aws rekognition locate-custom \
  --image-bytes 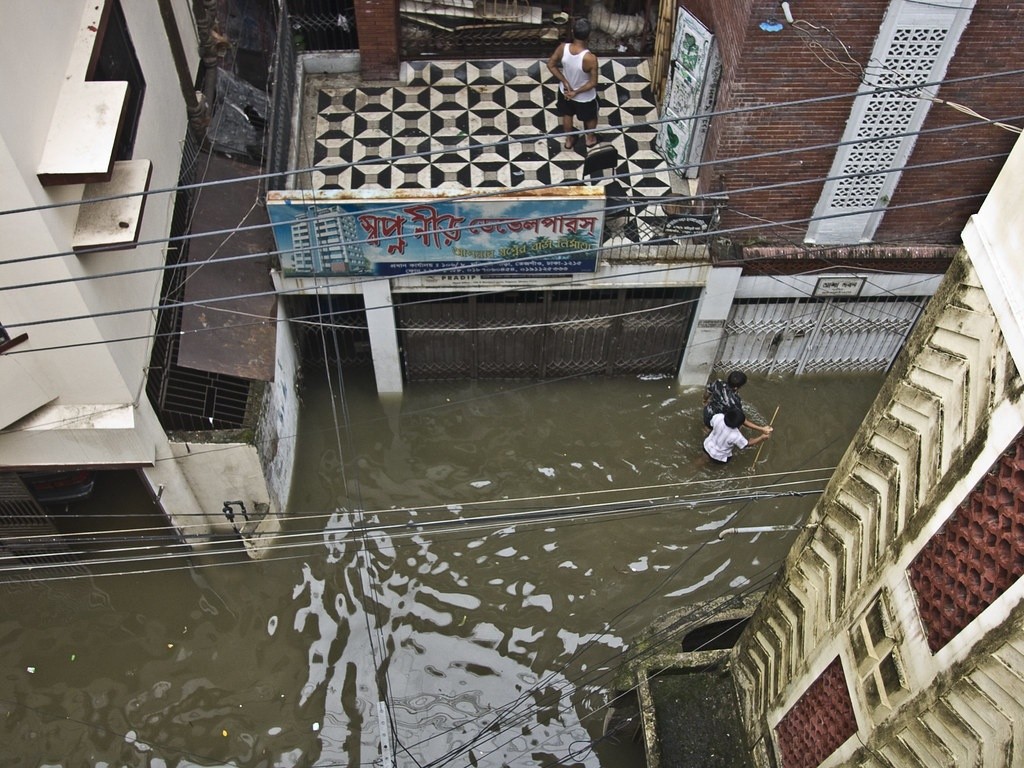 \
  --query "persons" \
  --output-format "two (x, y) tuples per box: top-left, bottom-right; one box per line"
(703, 408), (769, 464)
(701, 370), (773, 435)
(547, 18), (605, 149)
